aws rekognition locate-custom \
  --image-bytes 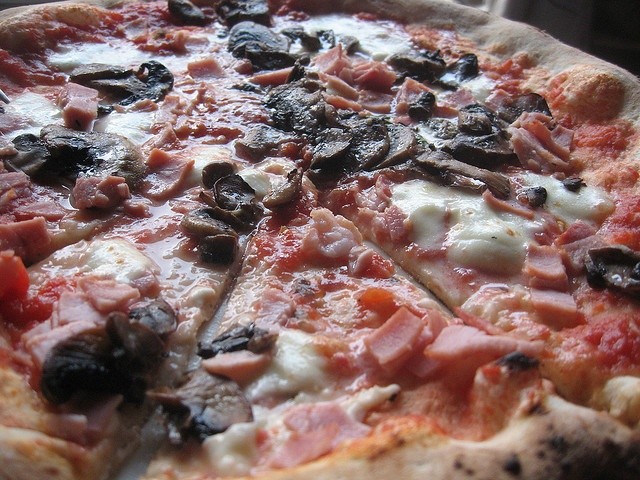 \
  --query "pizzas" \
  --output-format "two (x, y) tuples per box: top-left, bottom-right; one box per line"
(3, 0), (638, 480)
